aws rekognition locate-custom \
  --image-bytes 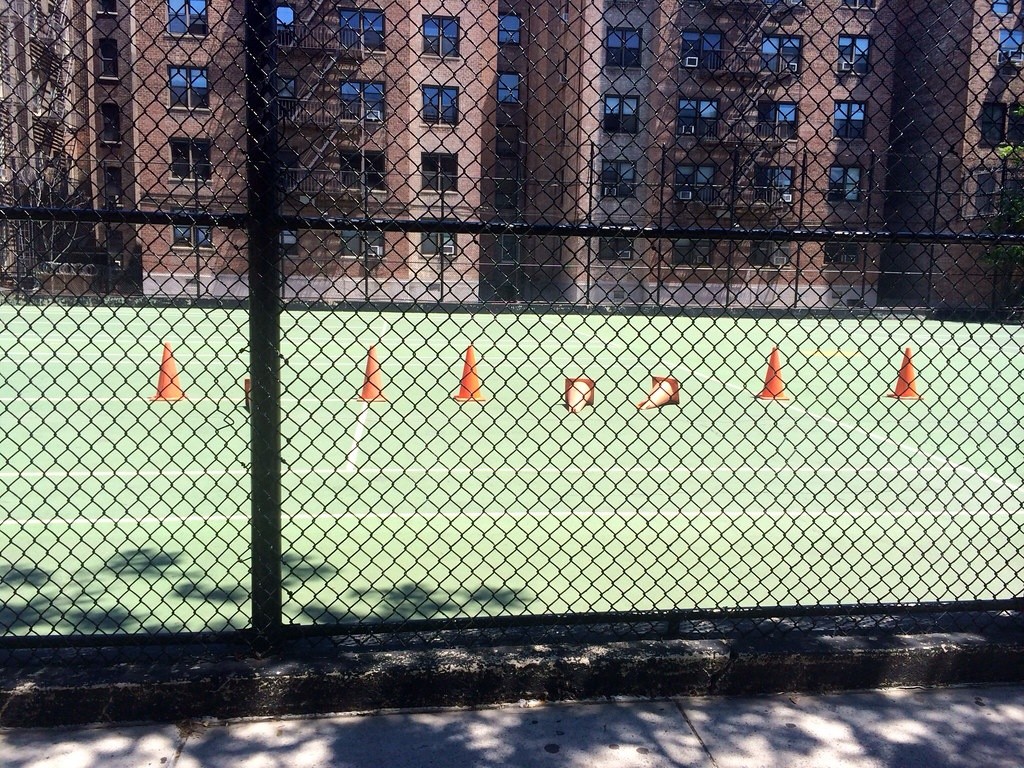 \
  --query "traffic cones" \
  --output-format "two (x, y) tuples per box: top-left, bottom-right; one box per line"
(565, 377), (595, 413)
(751, 348), (791, 402)
(244, 378), (252, 413)
(150, 343), (185, 401)
(451, 346), (485, 402)
(885, 348), (922, 399)
(636, 376), (680, 411)
(357, 345), (385, 404)
